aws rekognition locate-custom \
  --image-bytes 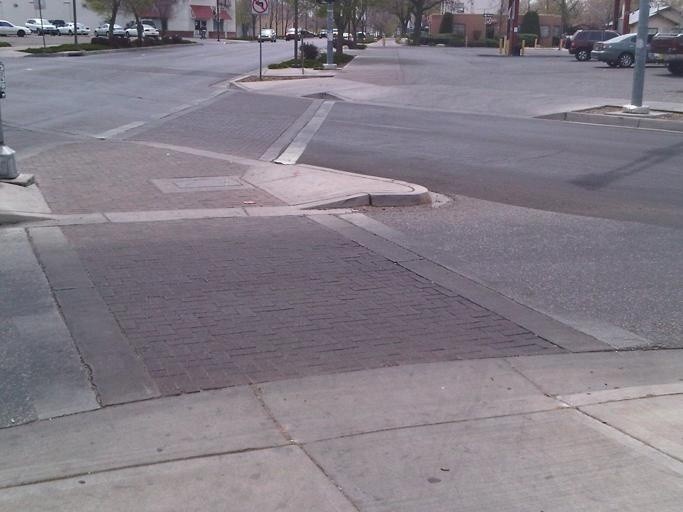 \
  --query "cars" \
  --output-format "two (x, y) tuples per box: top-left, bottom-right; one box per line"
(94, 19), (160, 39)
(562, 29), (683, 71)
(285, 28), (302, 41)
(319, 28), (366, 38)
(258, 29), (277, 43)
(0, 18), (90, 36)
